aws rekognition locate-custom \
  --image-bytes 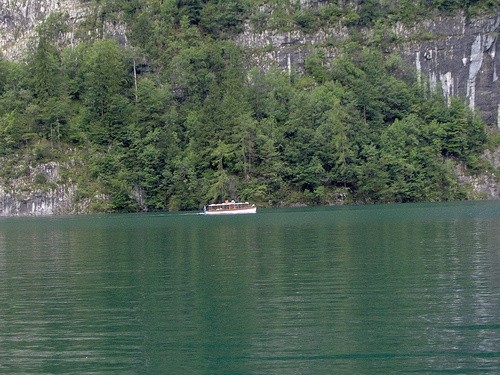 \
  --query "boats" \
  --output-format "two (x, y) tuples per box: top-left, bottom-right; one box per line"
(204, 199), (256, 215)
